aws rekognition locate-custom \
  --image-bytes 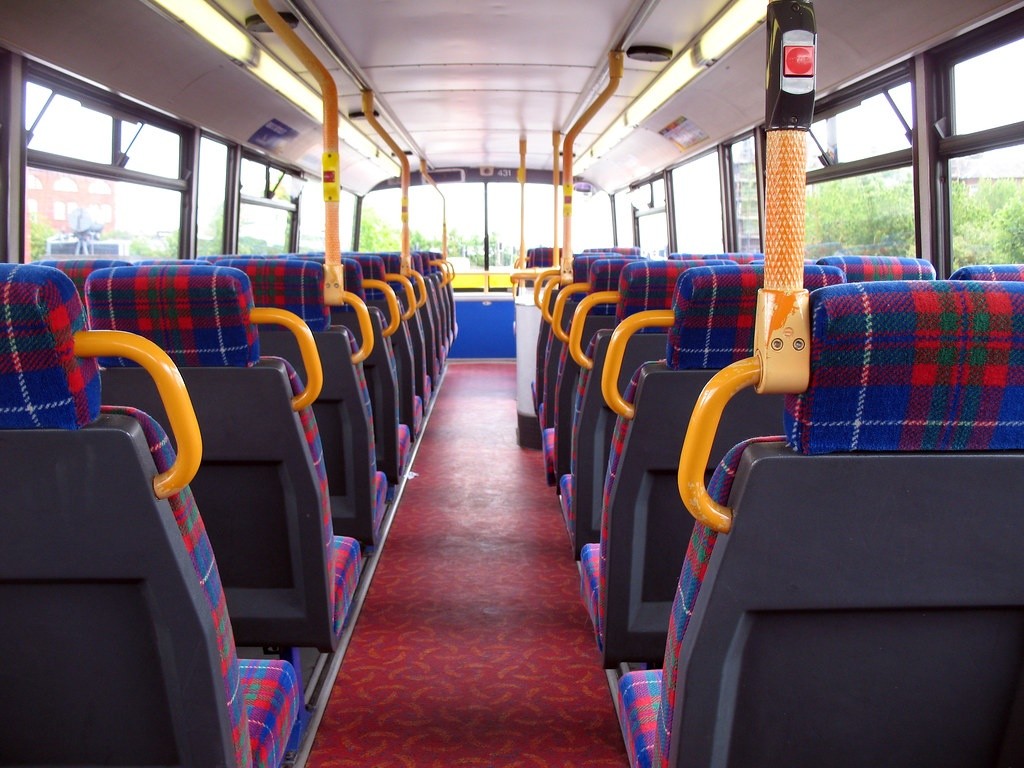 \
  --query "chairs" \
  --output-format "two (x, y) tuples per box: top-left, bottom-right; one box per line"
(0, 251), (461, 768)
(516, 248), (1024, 768)
